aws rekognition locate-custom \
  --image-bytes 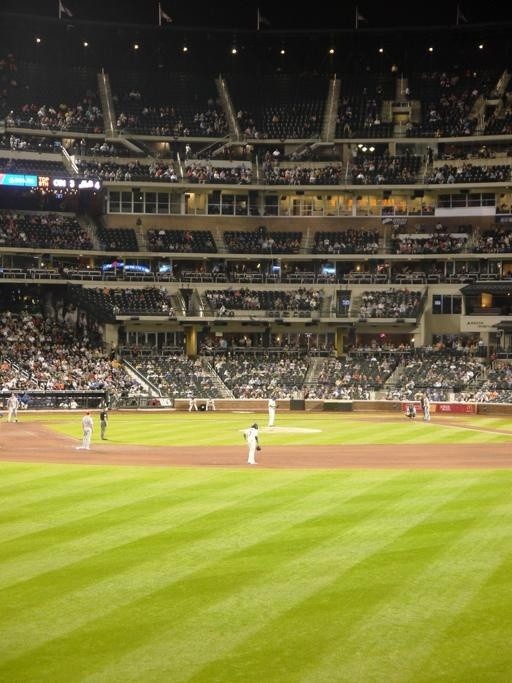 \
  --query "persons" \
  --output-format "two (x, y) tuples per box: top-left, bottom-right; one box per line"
(99, 407), (108, 439)
(81, 411), (93, 450)
(0, 69), (512, 425)
(243, 423), (259, 464)
